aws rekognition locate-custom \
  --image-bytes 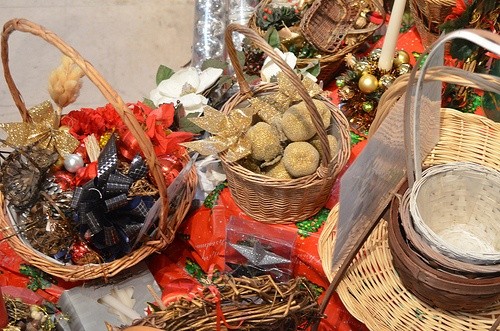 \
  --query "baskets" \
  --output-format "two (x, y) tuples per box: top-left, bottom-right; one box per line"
(301, 1), (364, 54)
(249, 0), (384, 84)
(318, 68), (500, 331)
(219, 25), (351, 225)
(409, 0), (497, 51)
(392, 29), (500, 308)
(0, 19), (197, 281)
